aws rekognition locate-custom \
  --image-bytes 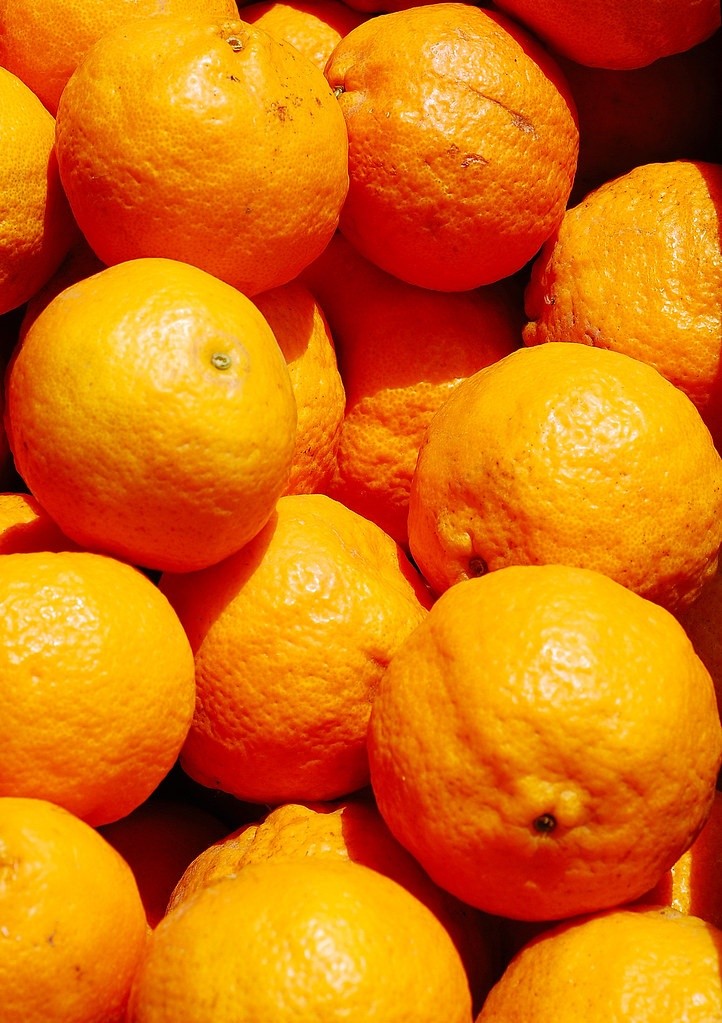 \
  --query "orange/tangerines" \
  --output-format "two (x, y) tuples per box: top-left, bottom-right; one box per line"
(0, 0), (722, 1023)
